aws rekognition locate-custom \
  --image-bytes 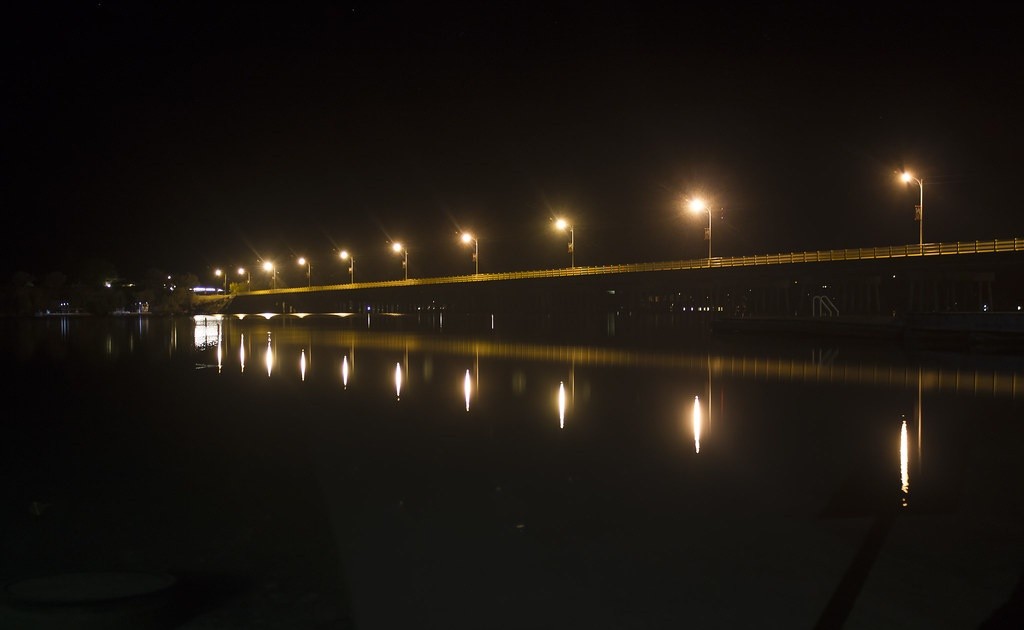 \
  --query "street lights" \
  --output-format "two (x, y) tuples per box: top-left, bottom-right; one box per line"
(240, 269), (250, 292)
(692, 203), (712, 258)
(216, 271), (226, 295)
(394, 244), (407, 279)
(301, 259), (310, 287)
(463, 235), (479, 274)
(341, 252), (353, 284)
(266, 265), (275, 289)
(558, 221), (573, 268)
(902, 174), (923, 253)
